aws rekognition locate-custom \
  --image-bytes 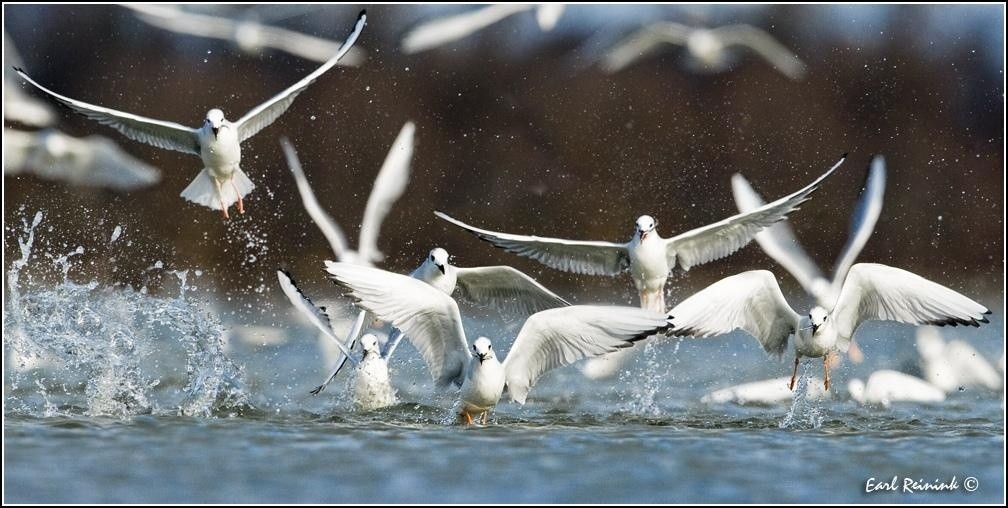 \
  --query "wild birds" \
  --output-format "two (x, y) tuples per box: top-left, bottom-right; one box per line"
(277, 121), (992, 429)
(601, 22), (807, 88)
(4, 4), (369, 219)
